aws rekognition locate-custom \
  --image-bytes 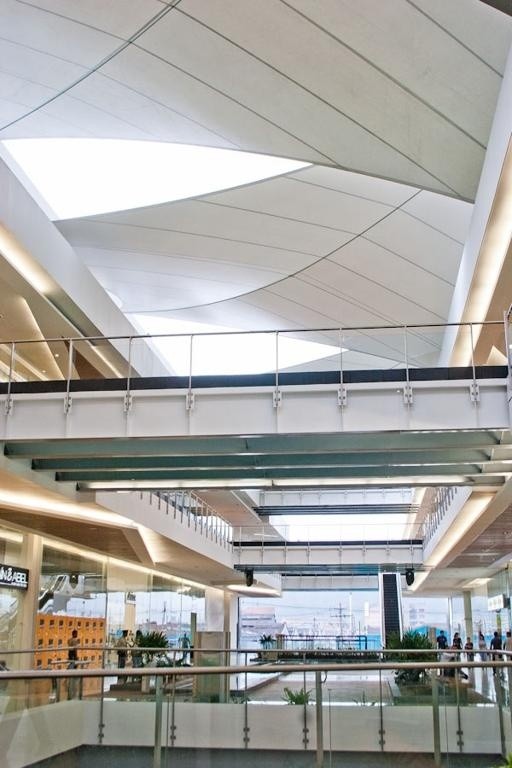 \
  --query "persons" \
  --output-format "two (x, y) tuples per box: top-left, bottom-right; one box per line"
(128, 630), (145, 667)
(63, 673), (81, 700)
(478, 634), (488, 670)
(452, 632), (463, 654)
(502, 631), (512, 672)
(116, 629), (129, 681)
(435, 630), (446, 651)
(67, 629), (80, 671)
(180, 633), (191, 657)
(464, 637), (475, 670)
(490, 632), (503, 676)
